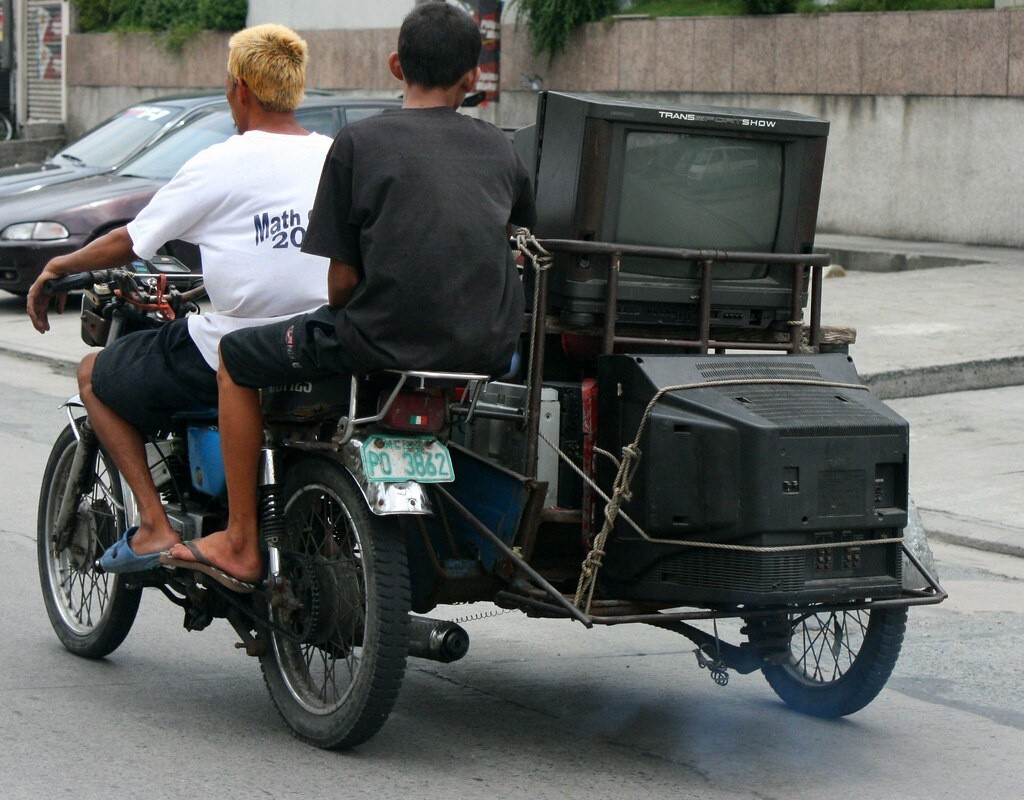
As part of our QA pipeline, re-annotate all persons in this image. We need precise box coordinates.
[157,2,539,594]
[26,23,336,576]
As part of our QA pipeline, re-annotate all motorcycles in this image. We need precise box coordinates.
[30,87,950,746]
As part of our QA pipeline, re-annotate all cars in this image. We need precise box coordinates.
[0,98,408,298]
[1,85,335,198]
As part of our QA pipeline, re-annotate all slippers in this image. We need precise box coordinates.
[159,538,256,594]
[100,525,159,573]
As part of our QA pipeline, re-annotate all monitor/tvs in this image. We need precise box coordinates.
[511,89,830,330]
[601,351,910,609]
